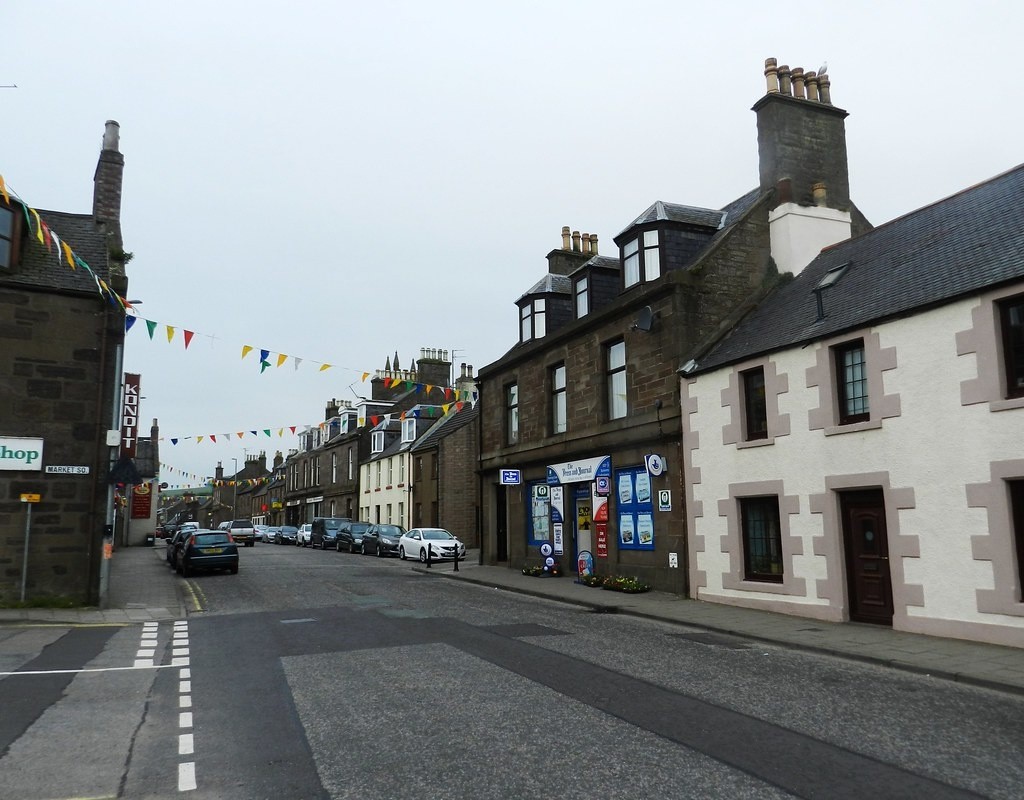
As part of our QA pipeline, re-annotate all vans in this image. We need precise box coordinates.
[312,517,352,549]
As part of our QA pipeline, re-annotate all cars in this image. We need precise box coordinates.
[218,521,229,529]
[276,526,299,544]
[263,527,279,543]
[335,520,374,553]
[253,525,269,542]
[156,527,164,537]
[164,525,239,576]
[296,523,313,547]
[361,524,408,557]
[399,528,466,563]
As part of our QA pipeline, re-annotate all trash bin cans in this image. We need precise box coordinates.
[146,533,155,547]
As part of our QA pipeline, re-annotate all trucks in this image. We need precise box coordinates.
[184,522,199,529]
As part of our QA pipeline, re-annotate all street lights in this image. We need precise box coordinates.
[231,458,237,519]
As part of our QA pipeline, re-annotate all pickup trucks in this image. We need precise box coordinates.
[226,519,255,547]
